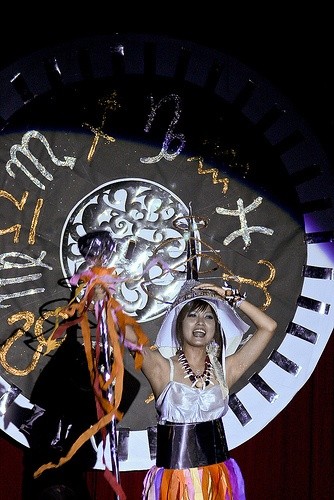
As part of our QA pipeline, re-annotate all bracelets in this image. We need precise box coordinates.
[224,289,248,309]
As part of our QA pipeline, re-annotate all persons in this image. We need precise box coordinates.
[94,281,277,500]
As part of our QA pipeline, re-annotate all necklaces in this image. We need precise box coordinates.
[175,349,215,390]
